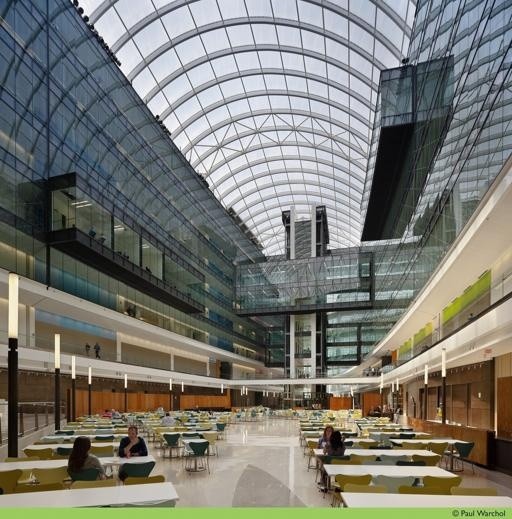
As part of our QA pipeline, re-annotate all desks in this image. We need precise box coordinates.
[0,407,512,508]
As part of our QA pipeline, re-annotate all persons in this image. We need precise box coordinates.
[118,425,148,482]
[85,342,91,358]
[127,303,133,316]
[94,342,101,359]
[111,408,120,417]
[319,430,346,494]
[146,266,152,273]
[66,435,107,481]
[103,409,112,416]
[317,425,346,449]
[160,412,174,426]
[366,365,381,377]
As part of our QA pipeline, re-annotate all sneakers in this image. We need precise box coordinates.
[321,486,328,493]
